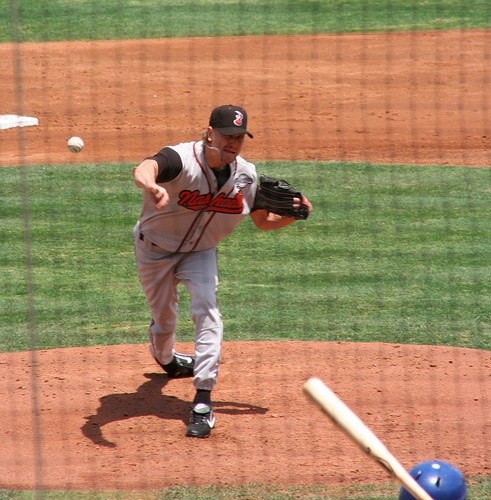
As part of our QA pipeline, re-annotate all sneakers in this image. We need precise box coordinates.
[185,402,216,439]
[156,350,197,378]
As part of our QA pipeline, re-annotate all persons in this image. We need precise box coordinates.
[134,104,312,438]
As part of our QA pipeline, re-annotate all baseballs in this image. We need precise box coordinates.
[68,137,84,154]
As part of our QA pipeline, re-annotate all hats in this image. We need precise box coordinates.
[209,104,254,140]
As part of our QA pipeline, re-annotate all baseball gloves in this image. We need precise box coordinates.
[251,173,310,223]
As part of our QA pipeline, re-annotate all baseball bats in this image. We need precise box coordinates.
[301,376,432,500]
